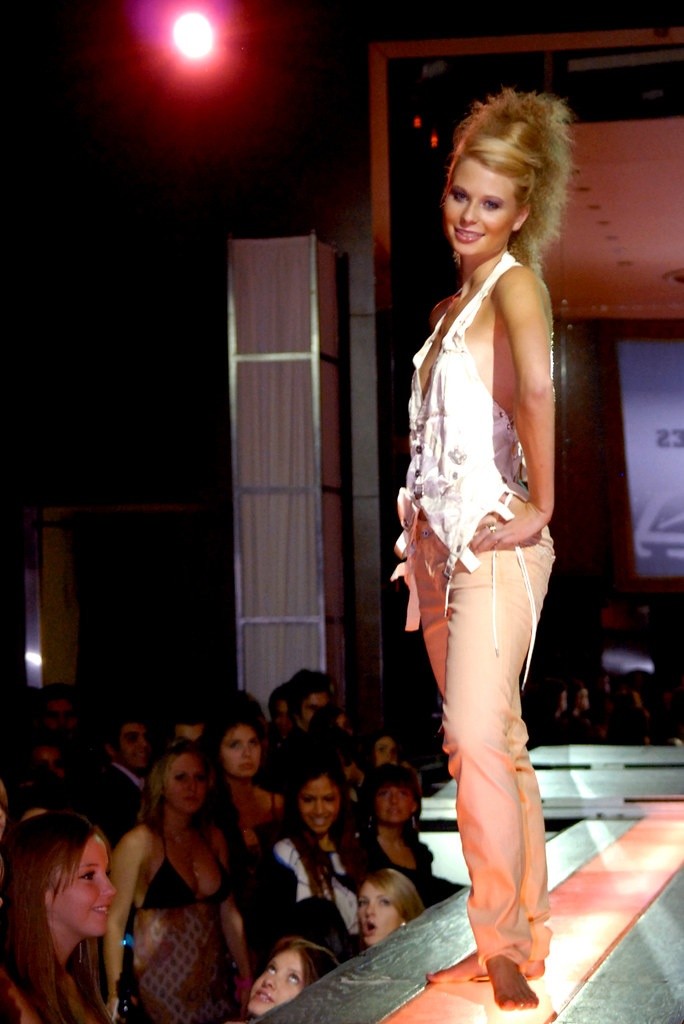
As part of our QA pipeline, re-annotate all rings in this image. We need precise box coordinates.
[489,524,496,533]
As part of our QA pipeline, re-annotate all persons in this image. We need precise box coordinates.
[0,665,436,1023]
[519,665,684,751]
[389,87,575,1011]
